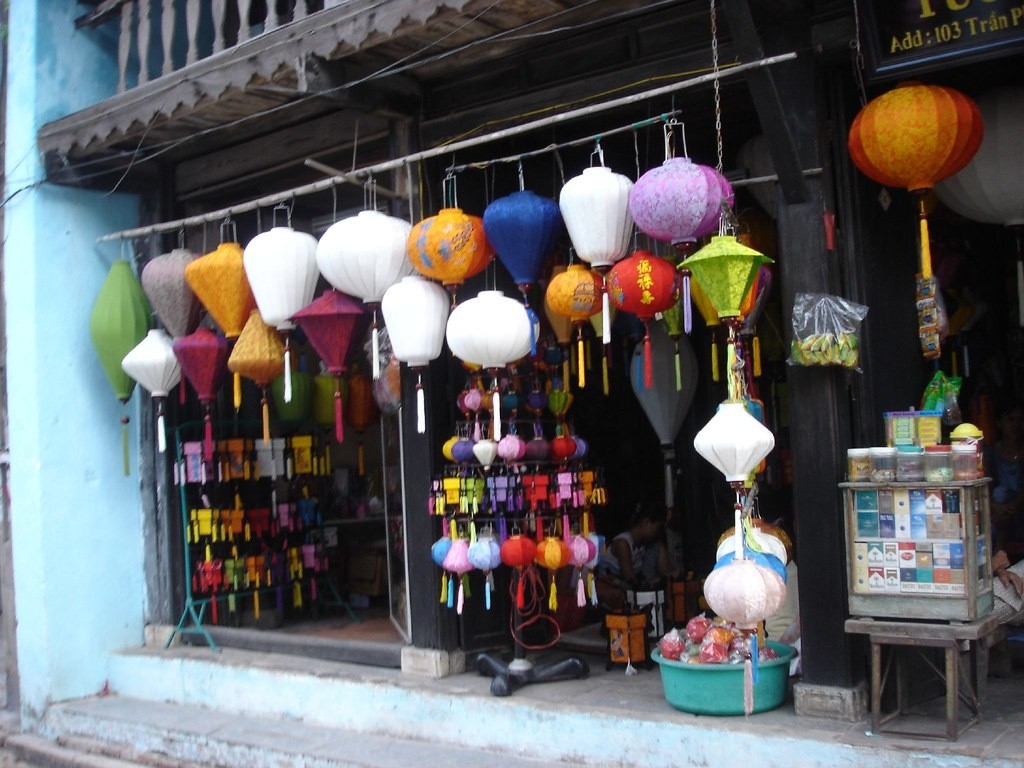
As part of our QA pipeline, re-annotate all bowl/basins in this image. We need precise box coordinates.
[651,640,798,715]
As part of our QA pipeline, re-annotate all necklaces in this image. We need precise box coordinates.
[1003,442,1020,462]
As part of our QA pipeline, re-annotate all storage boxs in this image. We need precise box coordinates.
[852,490,987,584]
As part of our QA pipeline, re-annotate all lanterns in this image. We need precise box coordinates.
[931,75,1024,327]
[88,156,793,715]
[924,241,992,381]
[847,80,982,279]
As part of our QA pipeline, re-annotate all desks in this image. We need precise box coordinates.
[322,512,369,525]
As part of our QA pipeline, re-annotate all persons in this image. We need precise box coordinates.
[596,508,673,640]
[983,401,1024,598]
